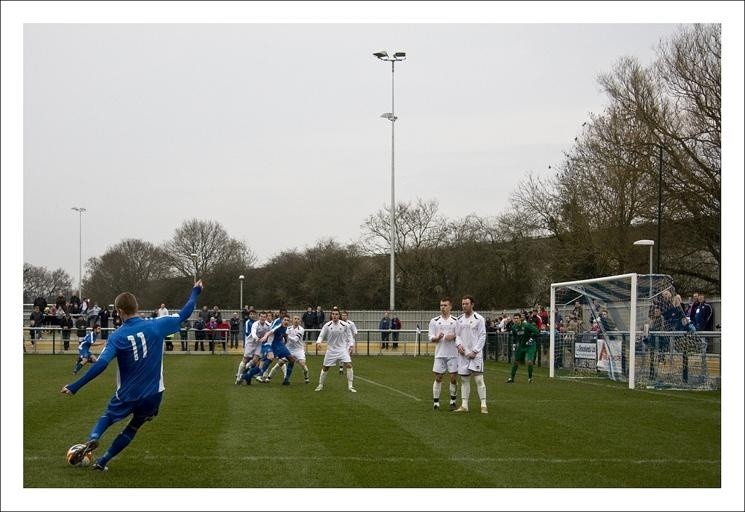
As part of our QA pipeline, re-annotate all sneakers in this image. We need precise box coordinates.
[235,370,358,393]
[504,377,514,383]
[453,405,469,413]
[69,438,100,466]
[527,378,535,384]
[449,402,456,411]
[480,406,488,414]
[91,464,109,472]
[433,404,439,411]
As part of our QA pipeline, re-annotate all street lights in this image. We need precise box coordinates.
[633,238,656,301]
[69,203,89,307]
[189,250,200,310]
[238,273,245,310]
[372,47,409,311]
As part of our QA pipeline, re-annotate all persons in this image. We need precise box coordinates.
[484,285,714,383]
[454,295,489,415]
[427,295,459,410]
[61,279,204,471]
[27,288,324,388]
[377,312,391,349]
[339,312,358,375]
[329,305,342,320]
[392,313,402,348]
[315,309,357,393]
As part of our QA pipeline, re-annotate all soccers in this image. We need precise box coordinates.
[68,444,92,467]
